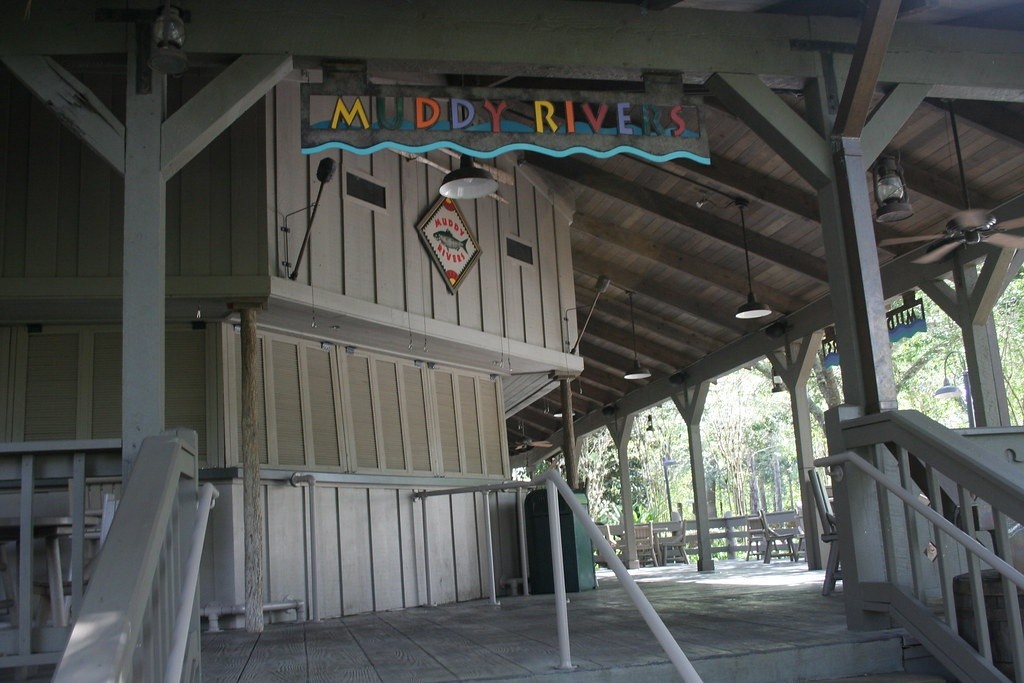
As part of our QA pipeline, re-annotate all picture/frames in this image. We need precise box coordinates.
[416,196,483,296]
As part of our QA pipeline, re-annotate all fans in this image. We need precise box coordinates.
[878,101,1024,265]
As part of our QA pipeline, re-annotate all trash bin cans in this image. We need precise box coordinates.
[524,490,598,596]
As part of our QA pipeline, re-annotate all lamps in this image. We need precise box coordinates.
[771,364,789,393]
[515,415,532,444]
[439,154,499,199]
[734,198,772,319]
[870,150,914,222]
[554,407,575,418]
[623,290,651,380]
[935,352,968,399]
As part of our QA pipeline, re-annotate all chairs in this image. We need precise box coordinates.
[745,510,799,564]
[595,520,691,567]
[807,469,845,596]
